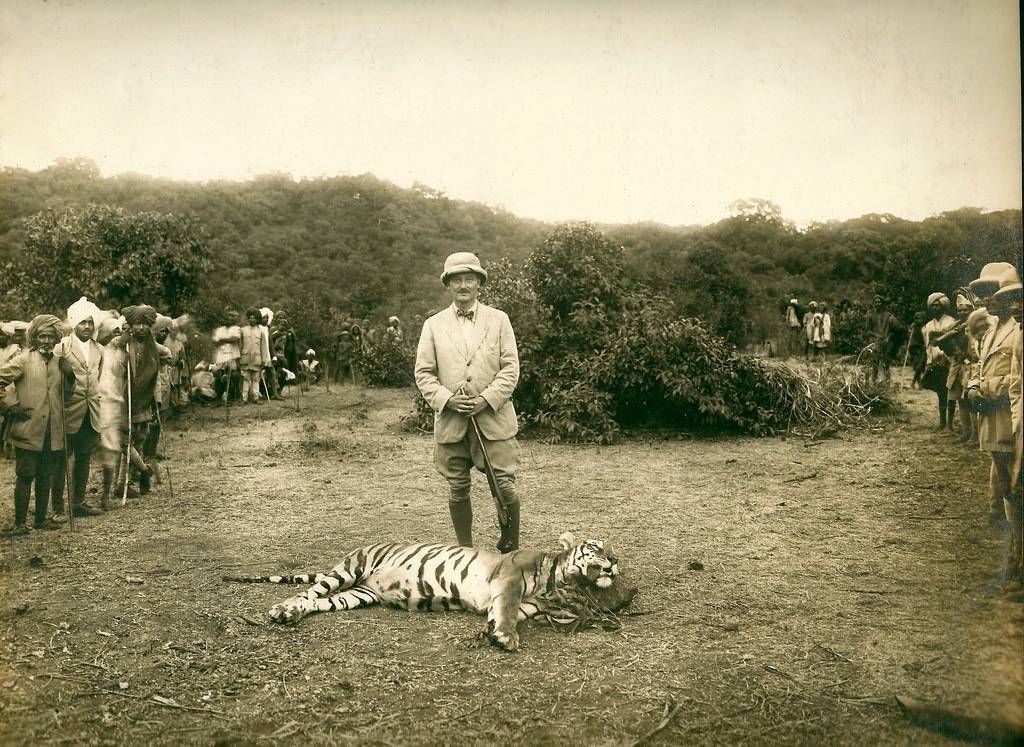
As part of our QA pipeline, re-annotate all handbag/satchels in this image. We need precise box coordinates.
[921,362,948,390]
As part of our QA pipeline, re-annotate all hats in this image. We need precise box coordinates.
[994,266,1022,296]
[441,252,487,288]
[969,262,1016,295]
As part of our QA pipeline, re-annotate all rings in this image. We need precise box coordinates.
[457,408,461,413]
[979,403,982,407]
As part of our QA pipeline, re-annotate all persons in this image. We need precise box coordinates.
[332,319,378,386]
[97,322,112,346]
[382,316,403,346]
[211,310,241,408]
[854,295,927,390]
[414,252,520,555]
[269,311,297,400]
[189,362,217,403]
[0,314,76,535]
[237,307,268,406]
[163,314,191,413]
[786,299,852,361]
[300,349,322,385]
[118,315,128,330]
[107,319,122,337]
[921,261,1024,593]
[257,307,285,401]
[52,295,104,522]
[413,315,422,326]
[0,320,28,453]
[99,302,173,510]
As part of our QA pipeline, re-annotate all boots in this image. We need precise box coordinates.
[499,497,519,552]
[447,498,472,546]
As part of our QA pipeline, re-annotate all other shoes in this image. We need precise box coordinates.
[271,394,284,400]
[956,432,969,441]
[918,384,923,390]
[985,513,1002,523]
[139,465,154,495]
[33,519,61,531]
[1005,556,1024,621]
[932,424,945,433]
[910,382,915,389]
[115,485,136,498]
[131,470,142,480]
[51,510,68,524]
[72,502,102,517]
[143,452,166,462]
[13,524,29,536]
[101,495,117,511]
[239,400,247,406]
[968,435,977,443]
[254,398,263,405]
[947,425,953,432]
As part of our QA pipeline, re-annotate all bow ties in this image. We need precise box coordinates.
[457,309,474,321]
